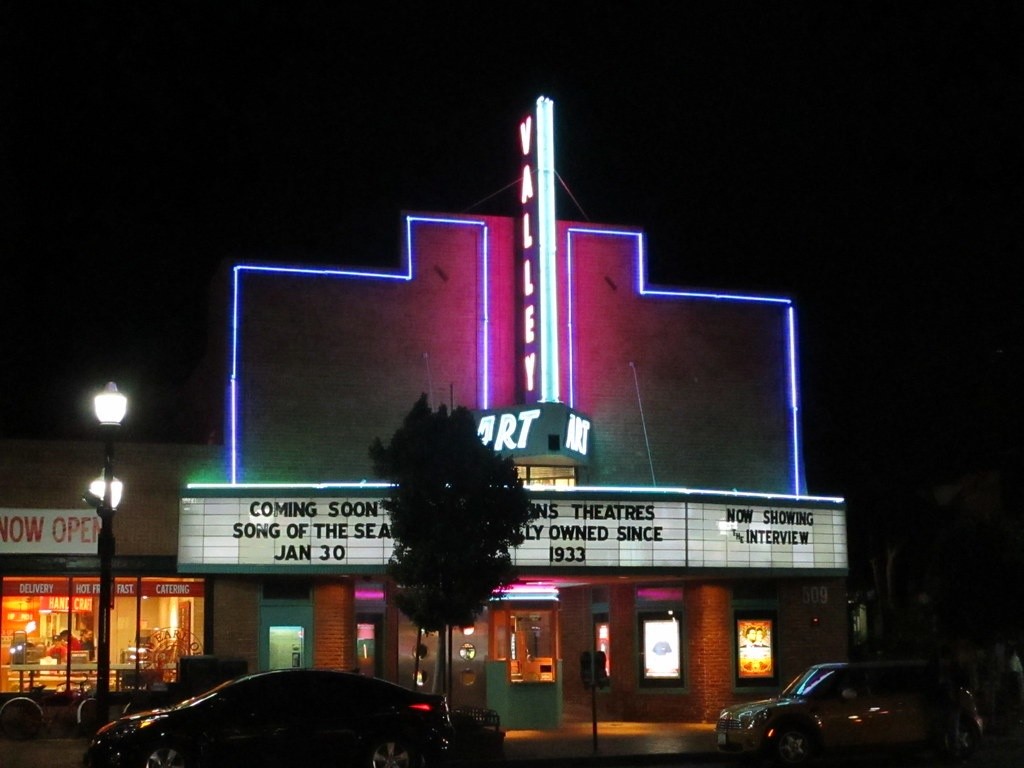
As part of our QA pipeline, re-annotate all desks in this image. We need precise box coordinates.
[10,663,176,693]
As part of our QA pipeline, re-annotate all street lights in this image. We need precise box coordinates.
[89,379,133,722]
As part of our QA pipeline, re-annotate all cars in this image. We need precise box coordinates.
[81,666,459,768]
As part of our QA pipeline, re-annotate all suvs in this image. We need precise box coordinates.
[713,662,987,768]
